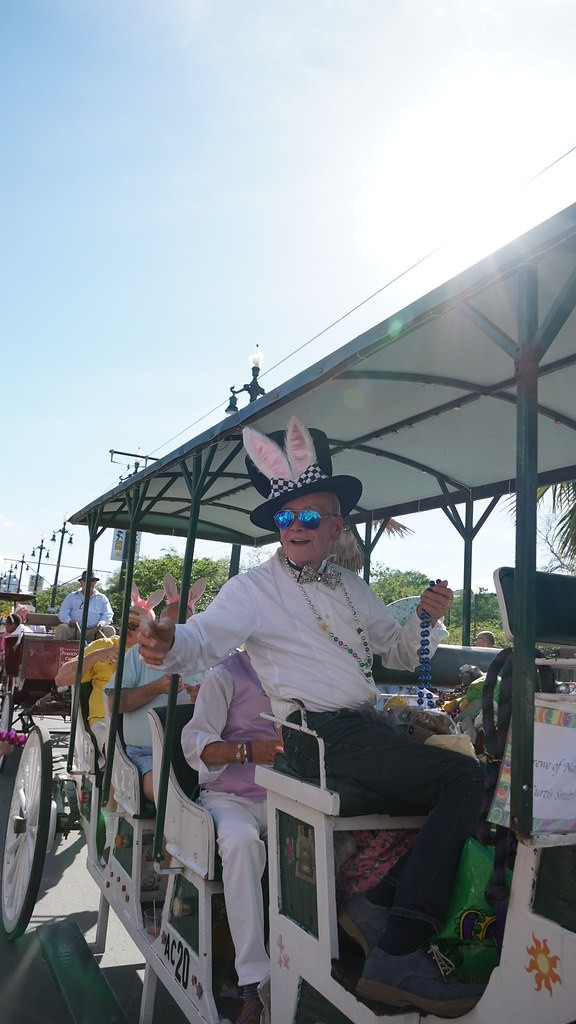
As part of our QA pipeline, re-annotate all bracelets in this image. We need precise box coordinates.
[236,740,253,765]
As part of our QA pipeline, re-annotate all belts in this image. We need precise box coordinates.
[283,712,329,741]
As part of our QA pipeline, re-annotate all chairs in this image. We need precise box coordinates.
[0,567,576,1022]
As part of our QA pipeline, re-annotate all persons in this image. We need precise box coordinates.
[104,602,207,803]
[55,571,116,639]
[137,491,500,1018]
[444,664,502,758]
[0,605,46,659]
[55,606,155,812]
[475,631,494,648]
[179,651,285,1024]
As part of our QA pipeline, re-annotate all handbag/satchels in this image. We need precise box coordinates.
[434,837,516,983]
[387,706,451,742]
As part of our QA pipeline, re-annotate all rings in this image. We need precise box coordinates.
[138,645,144,652]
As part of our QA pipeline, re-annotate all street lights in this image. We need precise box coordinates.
[119,446,144,594]
[14,555,29,594]
[31,536,49,595]
[0,564,16,593]
[48,516,73,614]
[225,344,267,581]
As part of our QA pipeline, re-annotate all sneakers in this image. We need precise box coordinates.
[338,892,390,958]
[357,943,487,1018]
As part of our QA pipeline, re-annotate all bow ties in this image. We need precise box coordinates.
[287,556,342,590]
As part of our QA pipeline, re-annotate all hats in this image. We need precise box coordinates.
[129,606,155,622]
[242,415,363,532]
[386,597,448,642]
[78,571,100,581]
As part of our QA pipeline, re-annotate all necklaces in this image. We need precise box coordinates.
[285,556,373,680]
[417,579,443,709]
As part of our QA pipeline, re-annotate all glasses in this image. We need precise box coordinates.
[473,638,487,642]
[273,508,339,530]
[118,621,139,631]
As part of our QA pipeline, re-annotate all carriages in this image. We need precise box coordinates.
[0,195,576,1024]
[0,589,119,772]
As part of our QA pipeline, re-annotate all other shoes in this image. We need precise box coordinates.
[173,899,192,919]
[235,997,263,1024]
